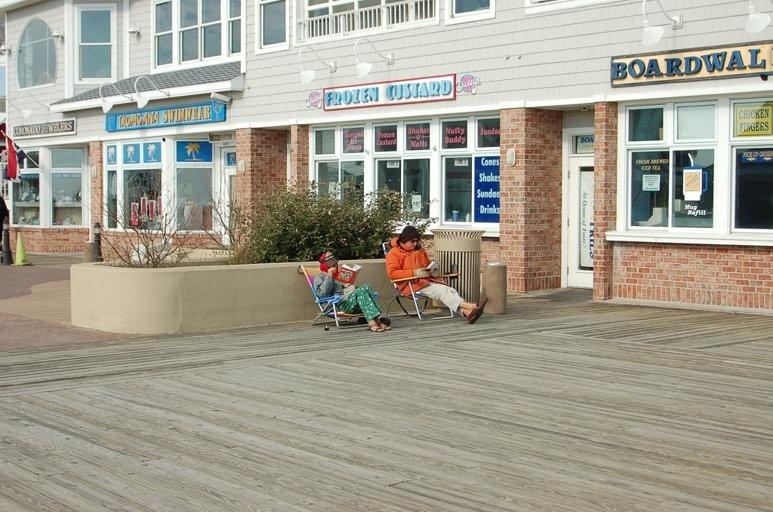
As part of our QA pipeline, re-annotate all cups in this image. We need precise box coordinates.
[451,210,461,222]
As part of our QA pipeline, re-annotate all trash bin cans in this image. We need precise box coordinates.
[83,241,95,262]
[482,262,507,315]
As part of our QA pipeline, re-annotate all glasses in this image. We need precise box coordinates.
[410,239,419,243]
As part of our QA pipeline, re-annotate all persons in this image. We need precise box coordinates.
[385,226,487,324]
[311,250,393,334]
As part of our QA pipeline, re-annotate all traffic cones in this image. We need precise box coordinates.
[11,232,33,266]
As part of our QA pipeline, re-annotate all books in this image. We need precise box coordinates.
[425,260,440,274]
[333,263,363,288]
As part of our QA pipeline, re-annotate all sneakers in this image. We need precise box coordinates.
[471,296,488,316]
[467,306,484,324]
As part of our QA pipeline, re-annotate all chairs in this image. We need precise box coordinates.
[296,258,387,331]
[379,240,464,326]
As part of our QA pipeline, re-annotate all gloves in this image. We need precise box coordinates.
[431,268,439,277]
[414,268,431,278]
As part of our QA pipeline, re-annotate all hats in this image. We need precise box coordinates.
[318,250,338,268]
[399,225,421,244]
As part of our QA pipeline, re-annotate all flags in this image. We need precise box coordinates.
[0,131,29,185]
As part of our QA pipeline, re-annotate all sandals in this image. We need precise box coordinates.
[378,322,392,330]
[368,324,385,332]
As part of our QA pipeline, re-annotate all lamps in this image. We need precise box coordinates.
[127,22,141,37]
[294,42,337,86]
[132,74,173,110]
[744,0,772,35]
[97,78,130,117]
[637,0,682,47]
[349,35,395,80]
[48,28,65,42]
[5,85,51,120]
[0,40,10,57]
